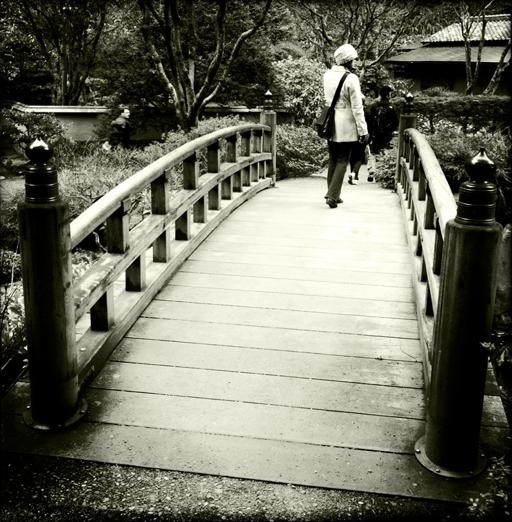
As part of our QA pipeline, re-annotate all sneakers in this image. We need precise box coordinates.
[324,168,374,208]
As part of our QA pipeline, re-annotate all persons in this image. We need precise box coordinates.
[102,101,133,150]
[364,84,400,182]
[347,96,376,186]
[321,41,371,211]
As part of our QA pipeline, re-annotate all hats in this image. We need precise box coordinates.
[334,44,358,66]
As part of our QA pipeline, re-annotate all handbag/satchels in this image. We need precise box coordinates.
[315,106,335,138]
